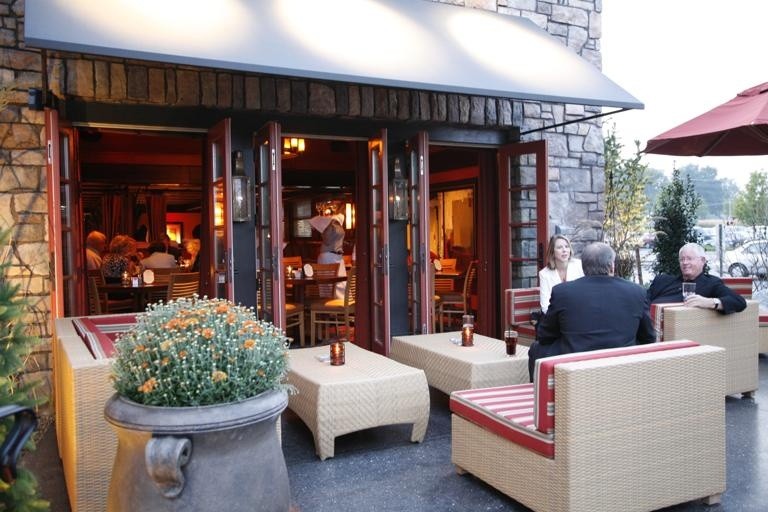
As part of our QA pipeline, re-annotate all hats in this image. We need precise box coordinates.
[715,240,768,279]
[644,233,658,250]
[690,226,768,249]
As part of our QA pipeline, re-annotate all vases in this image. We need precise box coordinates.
[103,291,301,409]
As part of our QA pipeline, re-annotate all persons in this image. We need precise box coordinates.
[646,242,747,315]
[537,234,585,316]
[84,211,354,303]
[527,241,657,383]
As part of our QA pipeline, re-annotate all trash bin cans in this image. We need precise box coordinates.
[710,297,720,310]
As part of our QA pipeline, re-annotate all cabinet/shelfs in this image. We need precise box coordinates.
[121,272,141,286]
[284,264,314,279]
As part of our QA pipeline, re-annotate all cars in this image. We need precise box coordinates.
[682,282,695,303]
[330,341,345,365]
[504,330,517,358]
[462,314,474,345]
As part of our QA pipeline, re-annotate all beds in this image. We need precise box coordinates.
[643,80,767,155]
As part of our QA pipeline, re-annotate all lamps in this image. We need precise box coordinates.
[447,338,730,512]
[55,330,149,512]
[644,294,761,400]
[716,274,768,358]
[166,270,201,302]
[145,266,185,283]
[63,272,103,318]
[503,285,547,347]
[247,246,479,349]
[51,309,147,459]
[84,267,113,314]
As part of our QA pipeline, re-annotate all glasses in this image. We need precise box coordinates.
[389,157,411,223]
[229,149,252,224]
[279,137,308,158]
